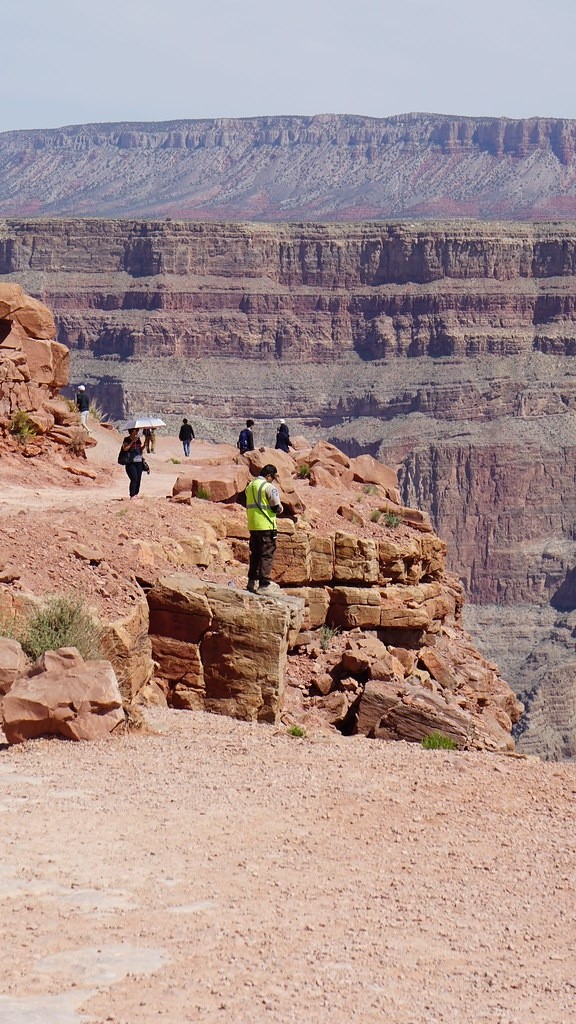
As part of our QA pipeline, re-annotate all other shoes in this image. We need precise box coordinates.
[246,580,260,591]
[256,585,282,597]
[147,450,150,453]
[151,449,154,451]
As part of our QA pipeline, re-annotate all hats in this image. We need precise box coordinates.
[280,419,285,423]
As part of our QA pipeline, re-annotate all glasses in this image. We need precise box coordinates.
[270,474,275,480]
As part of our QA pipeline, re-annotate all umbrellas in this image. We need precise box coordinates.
[124,417,166,431]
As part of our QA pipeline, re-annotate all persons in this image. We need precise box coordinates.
[237,420,254,454]
[179,419,195,457]
[143,429,157,454]
[245,464,284,589]
[275,419,293,452]
[118,428,150,499]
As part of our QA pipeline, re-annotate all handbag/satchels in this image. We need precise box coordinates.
[118,447,128,465]
